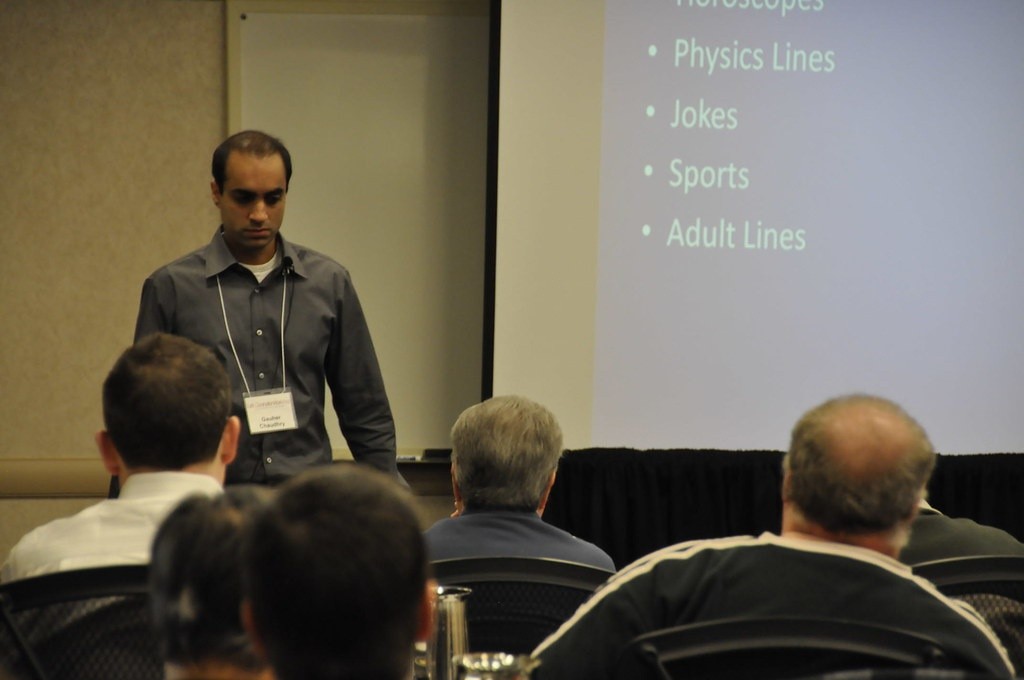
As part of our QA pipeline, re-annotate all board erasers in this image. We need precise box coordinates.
[421,447,453,461]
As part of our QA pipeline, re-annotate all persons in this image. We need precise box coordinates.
[0,332,241,584]
[132,130,399,486]
[148,465,432,680]
[528,395,1024,680]
[421,395,616,573]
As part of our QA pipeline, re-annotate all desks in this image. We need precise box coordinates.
[542,448,1024,572]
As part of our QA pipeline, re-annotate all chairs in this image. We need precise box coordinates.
[618,614,992,680]
[428,556,615,656]
[0,564,164,680]
[911,553,1024,680]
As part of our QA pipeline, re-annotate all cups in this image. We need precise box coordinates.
[426,585,474,680]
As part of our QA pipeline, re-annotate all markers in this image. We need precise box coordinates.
[396,457,416,460]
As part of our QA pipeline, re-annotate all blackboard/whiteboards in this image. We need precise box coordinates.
[225,2,490,466]
